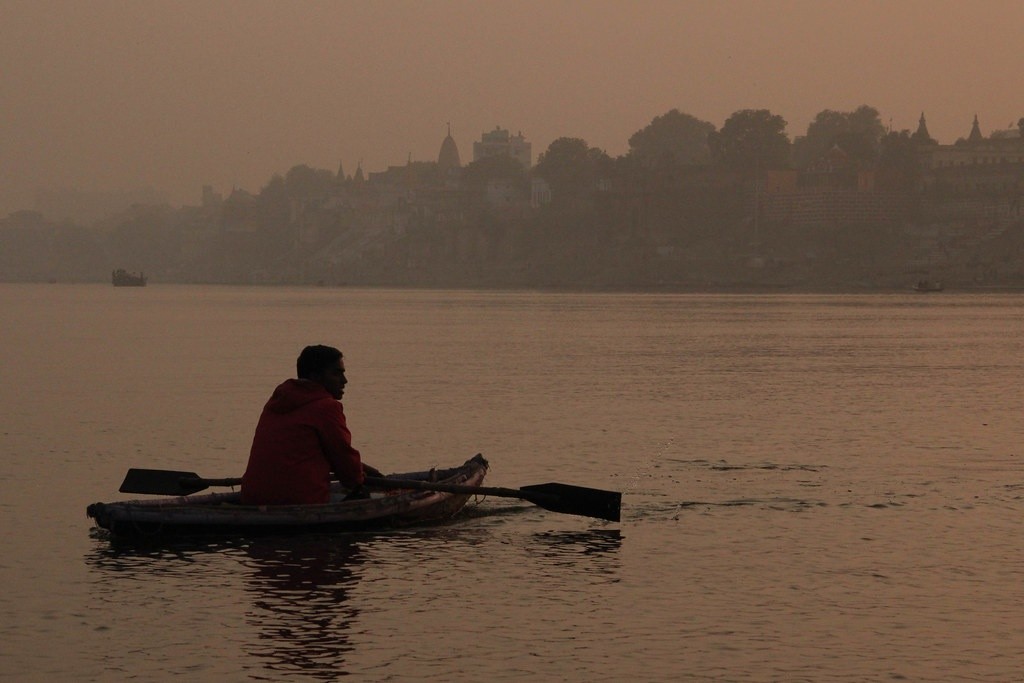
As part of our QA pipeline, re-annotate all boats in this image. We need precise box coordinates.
[112,268,148,286]
[911,285,940,291]
[86,453,492,536]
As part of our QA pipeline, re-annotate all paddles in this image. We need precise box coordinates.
[120,467,623,522]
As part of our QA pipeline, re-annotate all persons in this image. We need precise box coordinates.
[242,345,386,505]
